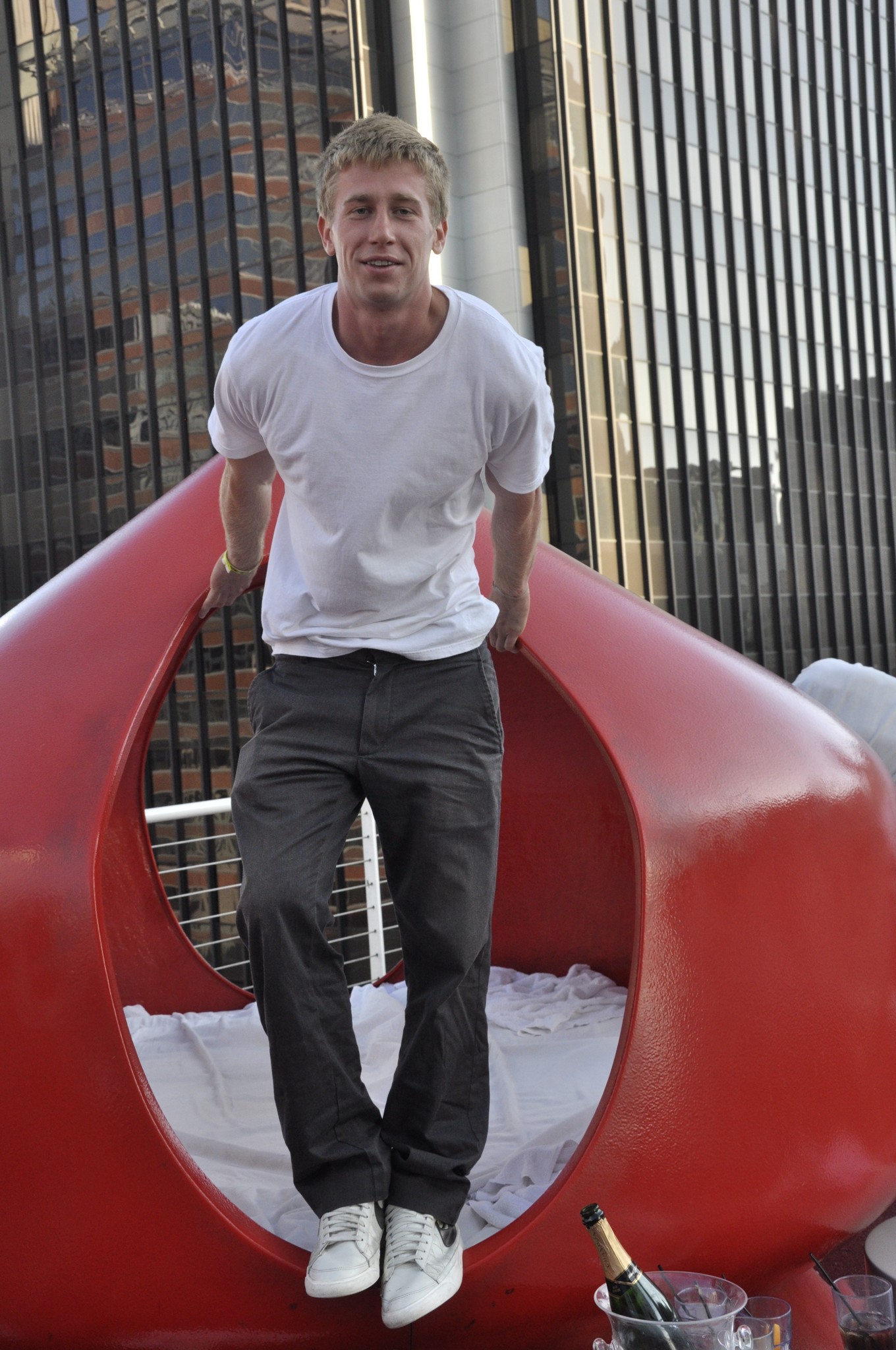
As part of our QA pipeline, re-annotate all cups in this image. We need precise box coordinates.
[733,1316,775,1349]
[738,1297,793,1350]
[831,1275,895,1349]
[675,1287,728,1328]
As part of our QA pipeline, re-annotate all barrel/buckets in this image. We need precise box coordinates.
[593,1272,747,1350]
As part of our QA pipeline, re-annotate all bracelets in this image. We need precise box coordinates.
[492,581,528,601]
[222,551,264,577]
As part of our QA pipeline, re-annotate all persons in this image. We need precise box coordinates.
[200,112,555,1330]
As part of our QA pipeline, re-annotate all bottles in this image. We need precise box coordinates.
[578,1202,697,1349]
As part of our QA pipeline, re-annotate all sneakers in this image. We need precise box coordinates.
[380,1204,464,1329]
[304,1200,384,1298]
